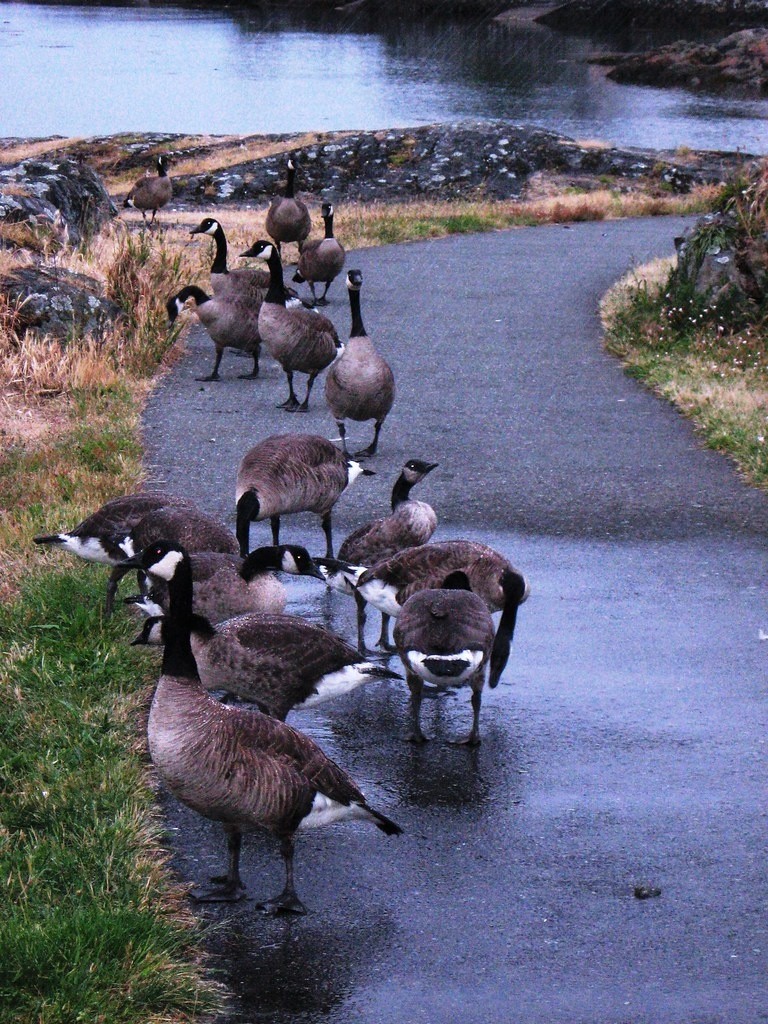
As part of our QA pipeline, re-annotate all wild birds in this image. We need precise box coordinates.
[30,431,532,918]
[120,154,173,227]
[163,152,397,461]
